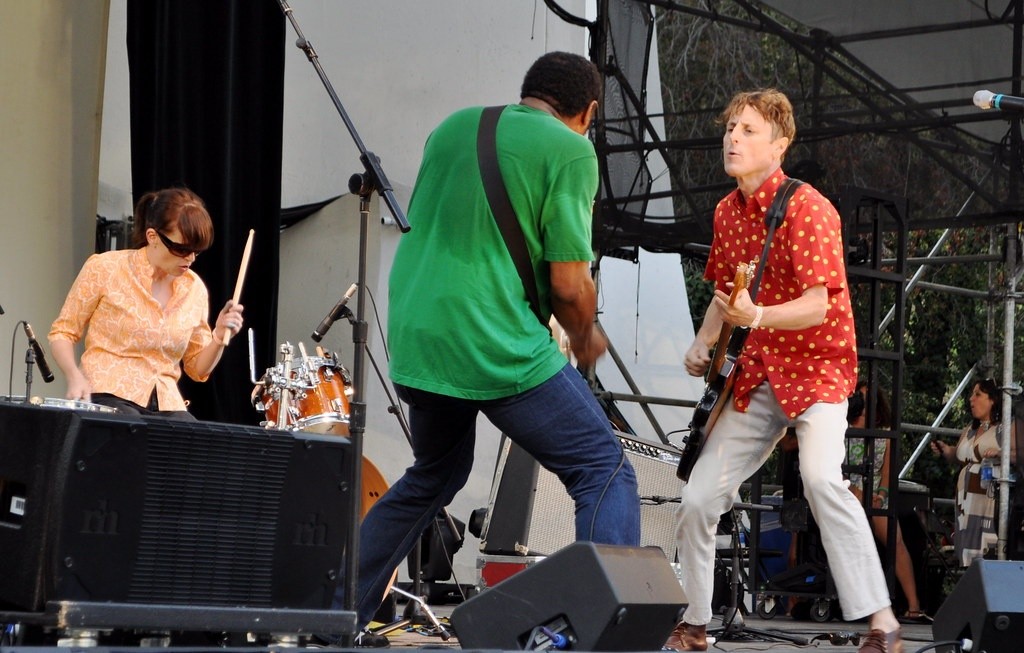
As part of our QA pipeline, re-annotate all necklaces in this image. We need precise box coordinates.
[979,421,991,431]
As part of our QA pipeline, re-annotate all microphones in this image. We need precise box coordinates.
[311,283,359,342]
[973,90,1024,111]
[24,321,55,383]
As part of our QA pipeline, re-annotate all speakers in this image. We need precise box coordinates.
[0,402,356,619]
[481,422,688,564]
[932,558,1024,653]
[450,540,690,652]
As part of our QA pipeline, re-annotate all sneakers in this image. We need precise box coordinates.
[310,626,391,648]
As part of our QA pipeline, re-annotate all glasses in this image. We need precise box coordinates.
[985,376,996,386]
[155,228,201,257]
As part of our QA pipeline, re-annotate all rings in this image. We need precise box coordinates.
[228,322,236,328]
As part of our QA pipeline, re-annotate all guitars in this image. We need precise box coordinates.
[676,255,759,483]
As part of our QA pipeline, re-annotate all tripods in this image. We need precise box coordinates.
[636,493,809,648]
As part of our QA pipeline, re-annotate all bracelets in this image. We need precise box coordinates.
[749,306,763,329]
[877,487,889,497]
[877,494,885,503]
[212,328,225,346]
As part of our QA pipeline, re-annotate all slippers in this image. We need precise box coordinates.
[904,610,920,618]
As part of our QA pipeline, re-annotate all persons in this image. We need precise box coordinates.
[778,380,920,618]
[930,380,1016,568]
[332,52,641,653]
[46,188,243,421]
[662,88,904,653]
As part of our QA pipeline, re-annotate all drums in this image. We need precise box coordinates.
[250,355,353,437]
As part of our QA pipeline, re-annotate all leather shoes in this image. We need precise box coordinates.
[664,622,708,651]
[856,626,904,653]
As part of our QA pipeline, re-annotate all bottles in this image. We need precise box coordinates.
[731,520,745,549]
[980,457,993,488]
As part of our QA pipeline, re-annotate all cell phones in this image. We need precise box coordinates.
[931,438,944,455]
